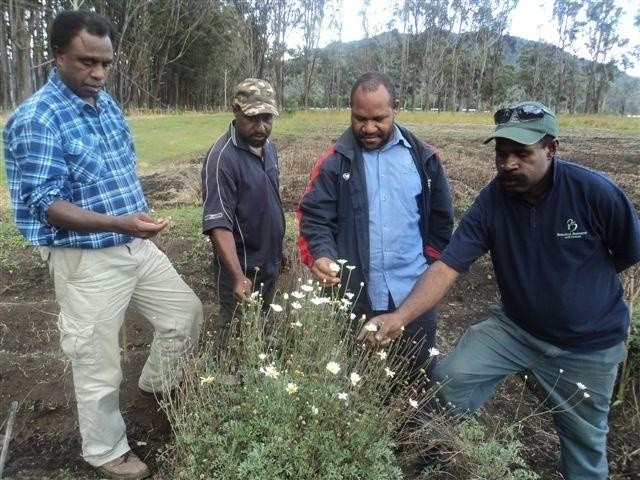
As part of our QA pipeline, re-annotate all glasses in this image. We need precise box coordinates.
[493,104,557,126]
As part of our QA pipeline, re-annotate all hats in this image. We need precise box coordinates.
[233,78,279,117]
[482,100,558,145]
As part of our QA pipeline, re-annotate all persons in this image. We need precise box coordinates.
[357,101,640,480]
[201,77,285,361]
[298,71,454,432]
[3,8,204,479]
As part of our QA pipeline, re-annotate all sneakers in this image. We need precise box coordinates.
[96,449,151,480]
